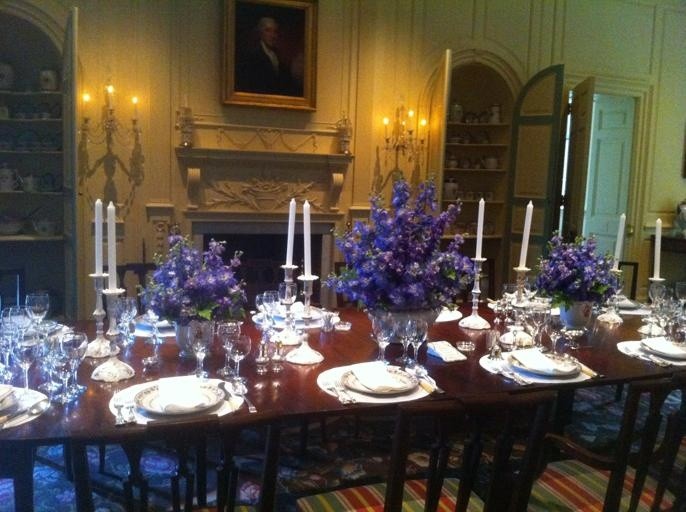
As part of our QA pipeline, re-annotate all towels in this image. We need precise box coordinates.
[511,345,579,375]
[158,372,205,411]
[280,298,309,319]
[644,335,685,358]
[351,359,410,393]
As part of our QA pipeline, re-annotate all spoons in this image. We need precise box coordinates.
[1,399,51,430]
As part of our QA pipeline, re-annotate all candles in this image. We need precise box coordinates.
[639,217,666,335]
[458,198,490,329]
[270,198,304,345]
[285,200,324,363]
[91,200,136,382]
[84,199,121,358]
[513,200,534,304]
[597,212,626,323]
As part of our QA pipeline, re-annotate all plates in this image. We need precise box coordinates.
[340,366,421,397]
[508,351,582,377]
[640,337,685,361]
[134,381,226,414]
[0,383,53,431]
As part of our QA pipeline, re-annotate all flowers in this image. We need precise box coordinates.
[527,230,624,312]
[136,234,247,324]
[320,172,487,312]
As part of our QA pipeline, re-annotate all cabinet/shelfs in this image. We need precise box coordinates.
[441,122,511,299]
[0,91,65,318]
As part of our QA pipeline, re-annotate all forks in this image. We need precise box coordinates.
[230,380,257,413]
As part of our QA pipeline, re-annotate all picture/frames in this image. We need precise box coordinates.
[222,0,318,111]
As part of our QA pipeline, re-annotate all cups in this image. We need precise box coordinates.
[675,280,685,310]
[543,317,568,353]
[255,293,274,332]
[9,332,40,396]
[60,332,88,396]
[112,297,138,347]
[457,325,479,352]
[531,303,547,338]
[263,290,280,313]
[186,319,215,378]
[227,333,252,385]
[372,316,396,367]
[278,282,298,312]
[392,315,417,366]
[35,336,66,394]
[405,319,428,368]
[8,305,33,339]
[24,292,50,332]
[648,283,667,316]
[217,319,241,378]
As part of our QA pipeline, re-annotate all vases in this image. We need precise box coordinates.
[176,323,213,358]
[561,301,592,330]
[380,311,435,344]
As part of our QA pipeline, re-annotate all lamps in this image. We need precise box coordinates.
[378,106,422,171]
[78,84,146,187]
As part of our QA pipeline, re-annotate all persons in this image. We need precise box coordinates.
[248,17,300,97]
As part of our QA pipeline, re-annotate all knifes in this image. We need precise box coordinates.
[215,381,237,412]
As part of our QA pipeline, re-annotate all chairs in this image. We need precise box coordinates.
[509,372,686,512]
[450,259,494,302]
[569,261,638,412]
[71,415,281,512]
[320,260,392,444]
[277,391,557,512]
[99,263,157,471]
[0,267,76,482]
[0,270,106,482]
